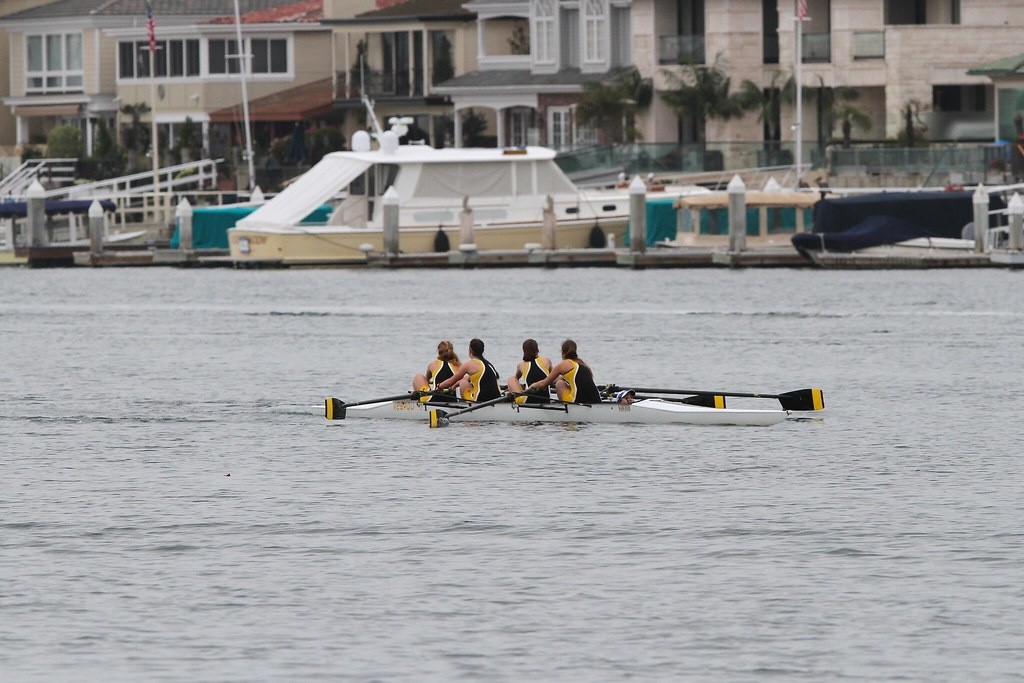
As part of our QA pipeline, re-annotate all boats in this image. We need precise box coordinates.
[308,394,794,426]
[0,155,120,215]
[791,183,1001,267]
[225,54,713,271]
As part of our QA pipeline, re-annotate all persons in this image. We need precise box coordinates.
[436,339,503,407]
[526,340,602,403]
[617,387,635,407]
[412,341,467,404]
[507,340,554,409]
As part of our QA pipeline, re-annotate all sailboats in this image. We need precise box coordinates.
[623,1,833,249]
[170,0,337,250]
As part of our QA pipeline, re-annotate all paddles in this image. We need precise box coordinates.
[546,386,727,410]
[324,387,450,421]
[428,388,534,429]
[493,383,827,411]
